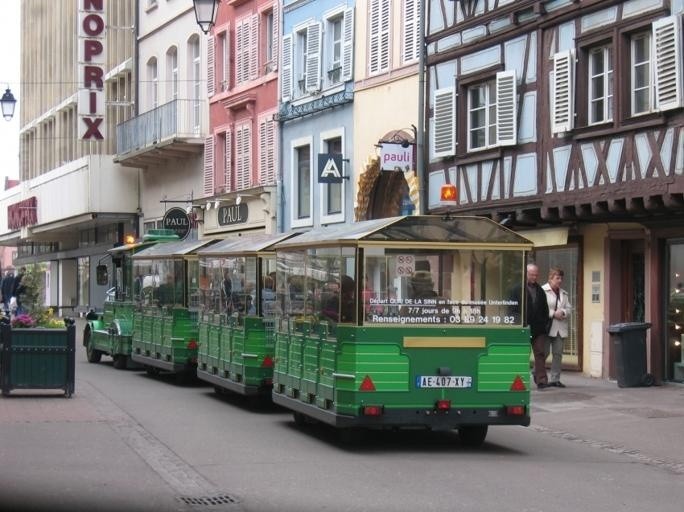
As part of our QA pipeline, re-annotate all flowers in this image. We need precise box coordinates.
[0,258,66,329]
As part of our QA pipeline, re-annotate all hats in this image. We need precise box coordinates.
[407,271,434,286]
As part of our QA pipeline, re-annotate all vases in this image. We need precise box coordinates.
[0,316,76,399]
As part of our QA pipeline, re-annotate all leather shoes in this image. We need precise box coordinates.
[550,381,565,387]
[537,383,549,387]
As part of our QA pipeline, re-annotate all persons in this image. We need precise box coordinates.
[541,268,573,388]
[223,271,276,315]
[321,274,385,324]
[401,270,443,321]
[159,272,174,303]
[0,271,15,311]
[10,272,18,310]
[11,267,30,315]
[508,263,549,389]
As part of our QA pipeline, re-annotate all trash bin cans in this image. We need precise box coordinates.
[606,321,655,389]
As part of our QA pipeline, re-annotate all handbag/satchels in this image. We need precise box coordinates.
[545,318,553,333]
